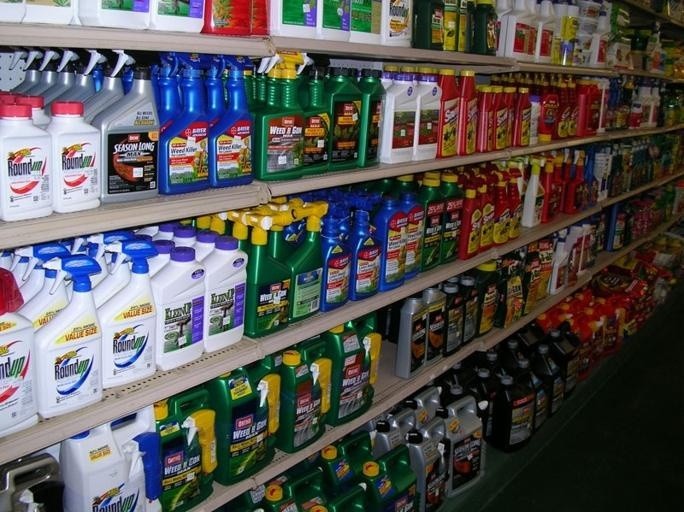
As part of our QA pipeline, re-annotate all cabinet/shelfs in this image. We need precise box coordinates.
[0,20,683,511]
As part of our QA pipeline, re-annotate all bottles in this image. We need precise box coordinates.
[0,0,613,66]
[553,203,628,294]
[369,167,553,381]
[302,66,684,176]
[150,221,248,373]
[0,90,103,223]
[535,289,625,384]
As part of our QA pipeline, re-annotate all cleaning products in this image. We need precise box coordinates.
[1,0,684,512]
